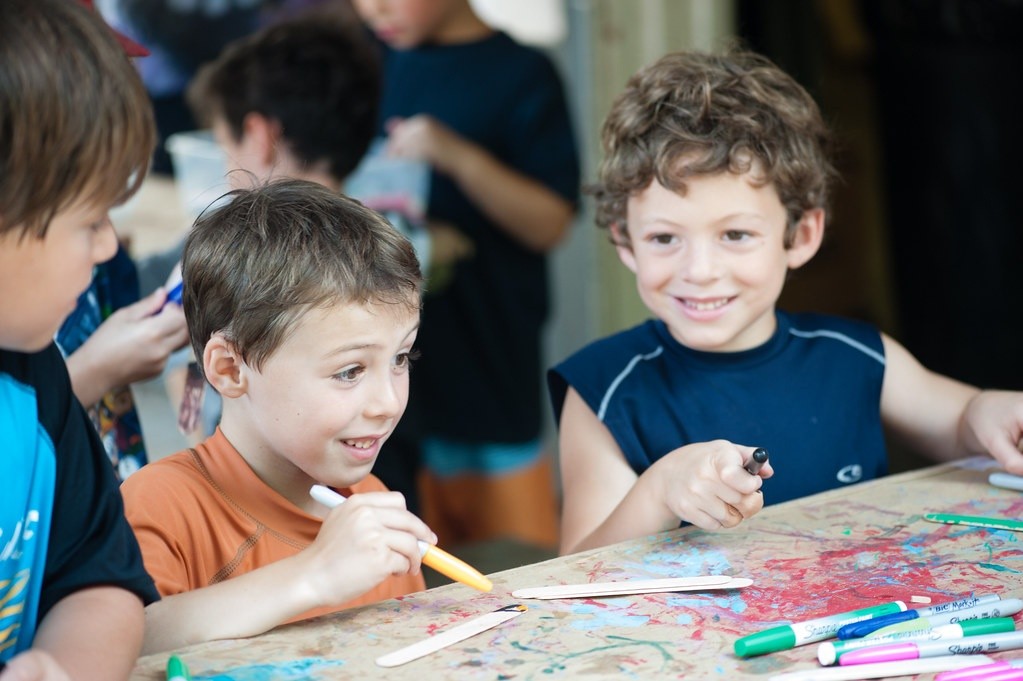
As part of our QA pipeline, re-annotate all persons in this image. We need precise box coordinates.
[1,0,159,681]
[97,0,583,556]
[118,180,438,659]
[556,50,1021,555]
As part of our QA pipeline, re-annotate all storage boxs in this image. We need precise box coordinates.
[167,127,428,234]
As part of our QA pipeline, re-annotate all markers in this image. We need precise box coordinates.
[309,481,494,594]
[745,447,768,476]
[165,654,187,681]
[731,592,1023,681]
[988,472,1023,492]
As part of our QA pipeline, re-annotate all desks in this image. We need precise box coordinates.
[136,452,1023,681]
